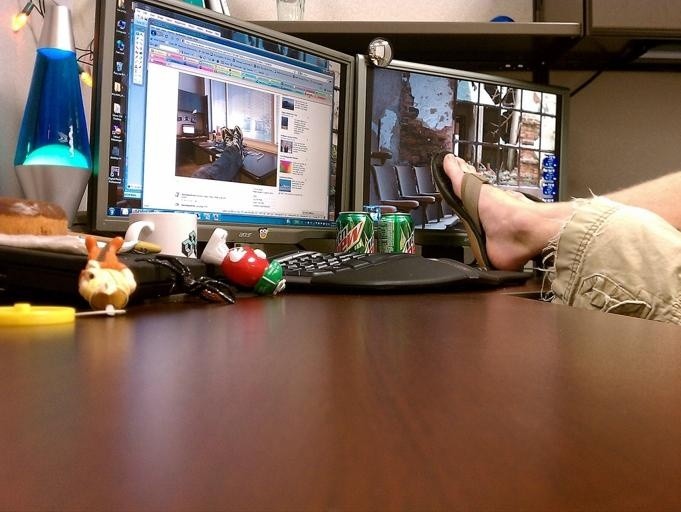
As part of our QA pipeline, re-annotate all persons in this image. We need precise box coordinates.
[191,126,242,181]
[434,151,681,332]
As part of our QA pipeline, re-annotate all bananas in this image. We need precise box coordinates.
[267,250,534,296]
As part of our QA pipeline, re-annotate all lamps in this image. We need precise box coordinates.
[412,166,454,218]
[372,165,435,230]
[396,165,442,224]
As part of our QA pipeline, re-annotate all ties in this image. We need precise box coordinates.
[85,4,355,242]
[356,52,571,262]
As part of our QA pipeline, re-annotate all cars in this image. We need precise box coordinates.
[123,211,197,259]
[276,0,305,21]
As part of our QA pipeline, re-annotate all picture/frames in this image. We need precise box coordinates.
[191,141,277,185]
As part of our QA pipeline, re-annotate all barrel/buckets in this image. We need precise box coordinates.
[432,151,494,273]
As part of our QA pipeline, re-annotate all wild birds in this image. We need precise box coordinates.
[181,123,197,139]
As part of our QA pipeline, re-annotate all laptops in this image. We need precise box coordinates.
[1,199,67,236]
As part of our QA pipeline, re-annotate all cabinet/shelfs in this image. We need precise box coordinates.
[336,210,375,255]
[377,212,416,256]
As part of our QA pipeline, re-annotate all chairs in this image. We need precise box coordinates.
[221,125,232,147]
[233,126,243,159]
